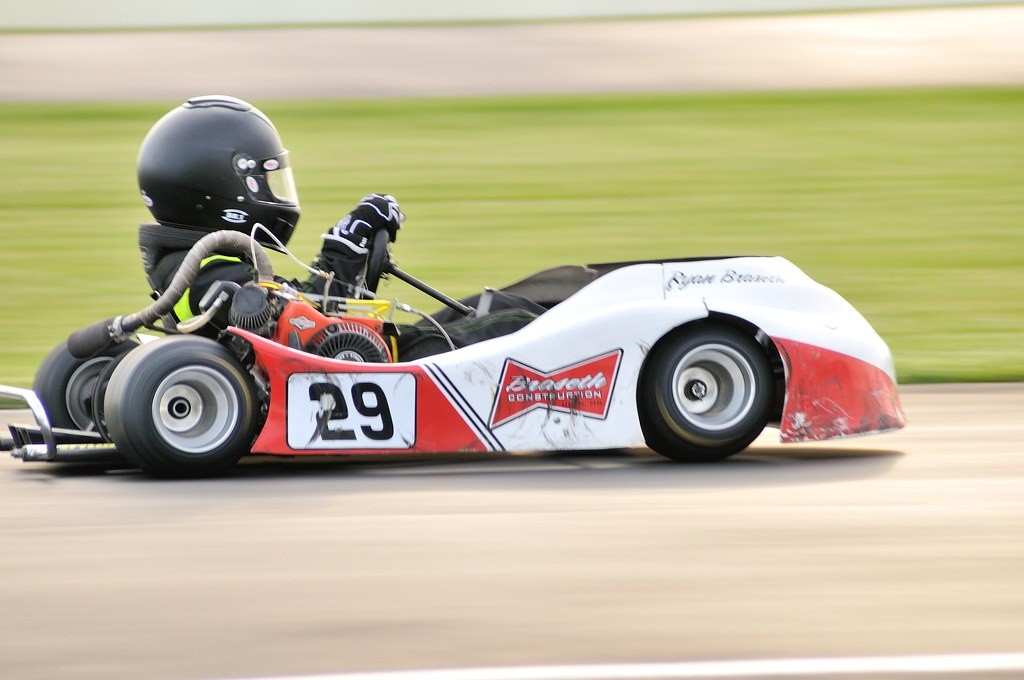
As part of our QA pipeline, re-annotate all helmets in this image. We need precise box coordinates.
[137,96,300,248]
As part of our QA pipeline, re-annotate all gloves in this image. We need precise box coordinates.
[322,194,403,259]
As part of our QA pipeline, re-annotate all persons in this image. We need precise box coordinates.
[137,95,540,364]
